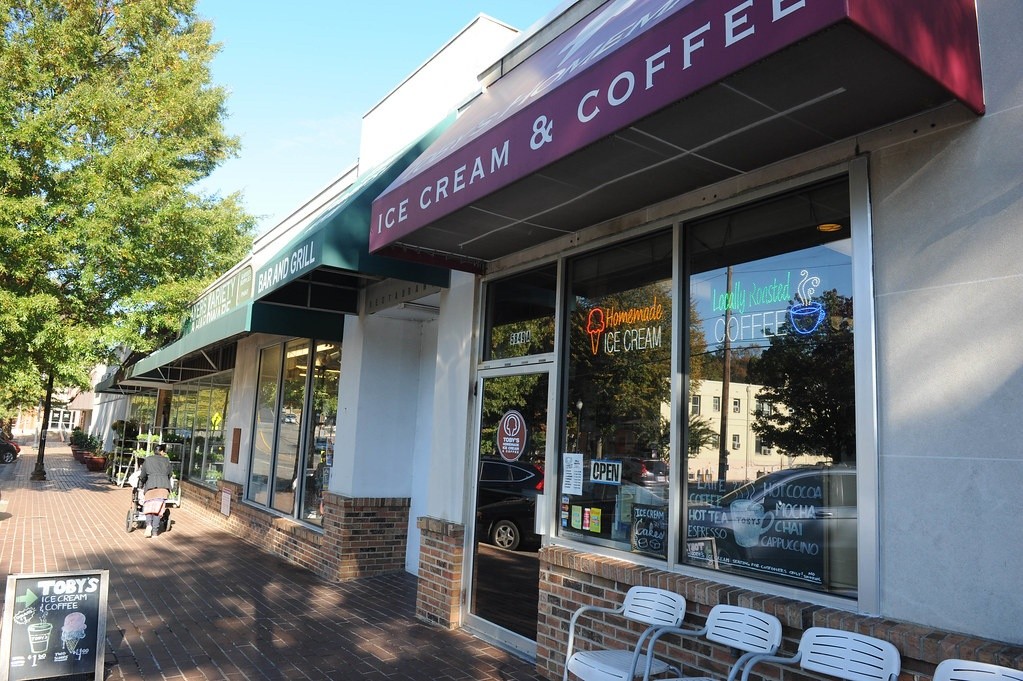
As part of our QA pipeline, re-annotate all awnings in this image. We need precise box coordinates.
[67,389,92,411]
[254,0,987,316]
[95,373,157,397]
[121,302,345,385]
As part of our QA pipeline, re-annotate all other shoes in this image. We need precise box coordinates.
[152,532,158,538]
[144,525,152,538]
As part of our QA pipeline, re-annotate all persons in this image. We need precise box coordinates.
[139,445,173,537]
[307,452,333,519]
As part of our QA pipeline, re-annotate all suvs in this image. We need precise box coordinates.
[652,462,857,589]
[478,458,543,511]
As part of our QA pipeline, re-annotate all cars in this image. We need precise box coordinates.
[477,479,670,551]
[284,414,297,423]
[315,437,335,453]
[0,436,16,463]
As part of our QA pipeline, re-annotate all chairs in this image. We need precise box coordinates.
[563,586,1022,681]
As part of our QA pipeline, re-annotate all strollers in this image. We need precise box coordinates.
[125,475,171,532]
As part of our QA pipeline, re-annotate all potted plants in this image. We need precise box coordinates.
[69,426,106,472]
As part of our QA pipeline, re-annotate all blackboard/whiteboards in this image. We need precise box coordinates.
[0,569,109,681]
[629,501,668,561]
[680,464,831,593]
